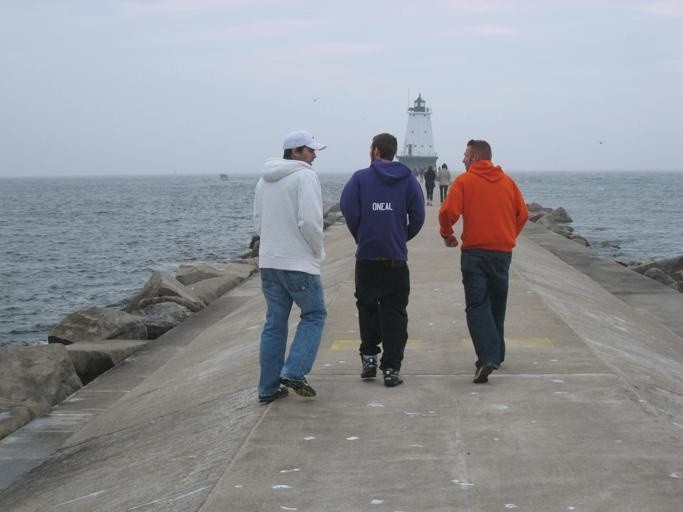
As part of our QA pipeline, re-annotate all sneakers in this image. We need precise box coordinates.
[472,361,495,385]
[359,354,378,379]
[279,376,318,399]
[383,368,403,387]
[258,386,289,404]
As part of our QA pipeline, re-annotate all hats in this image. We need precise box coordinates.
[282,130,327,155]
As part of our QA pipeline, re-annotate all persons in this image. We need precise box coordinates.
[339,133,425,386]
[253,132,327,405]
[413,163,451,206]
[438,139,528,383]
